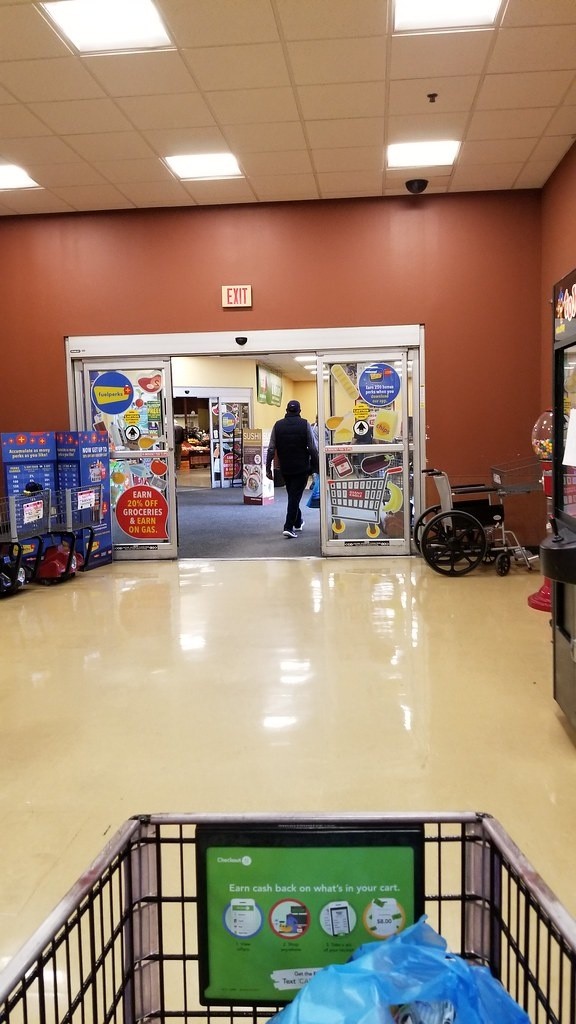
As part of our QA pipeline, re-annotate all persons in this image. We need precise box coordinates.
[265,400,319,538]
[309,413,330,490]
[173,419,183,471]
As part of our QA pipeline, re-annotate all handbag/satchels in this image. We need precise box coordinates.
[265,915,532,1024]
[307,473,320,508]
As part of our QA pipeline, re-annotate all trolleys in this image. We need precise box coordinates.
[328,467,402,539]
[353,453,413,501]
[0,813,576,1024]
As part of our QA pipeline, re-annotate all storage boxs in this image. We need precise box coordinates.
[180,460,189,470]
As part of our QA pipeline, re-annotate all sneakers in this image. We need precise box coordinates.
[283,529,297,537]
[296,519,304,530]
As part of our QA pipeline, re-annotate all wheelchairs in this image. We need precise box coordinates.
[414,456,542,577]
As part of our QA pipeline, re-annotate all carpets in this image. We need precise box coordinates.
[112,488,415,558]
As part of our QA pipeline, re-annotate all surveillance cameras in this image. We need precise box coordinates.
[406,178,429,193]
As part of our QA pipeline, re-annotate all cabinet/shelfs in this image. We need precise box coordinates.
[180,428,210,468]
[540,269,576,726]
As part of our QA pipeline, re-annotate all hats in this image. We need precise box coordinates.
[287,400,300,410]
